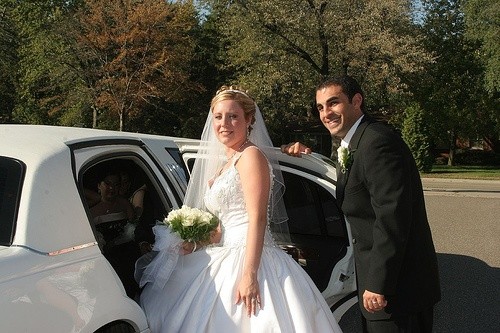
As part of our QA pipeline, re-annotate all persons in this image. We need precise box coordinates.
[88,172,137,268]
[140,85,342,333]
[280,75,440,333]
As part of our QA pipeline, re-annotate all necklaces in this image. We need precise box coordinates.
[217,139,250,176]
[99,197,116,214]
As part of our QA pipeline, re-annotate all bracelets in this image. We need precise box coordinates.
[191,241,197,253]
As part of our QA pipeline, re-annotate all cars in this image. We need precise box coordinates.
[0,124,363,333]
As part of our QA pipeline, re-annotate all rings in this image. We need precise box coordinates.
[372,302,378,304]
[251,298,256,301]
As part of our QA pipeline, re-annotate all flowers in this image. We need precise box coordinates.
[163,205,219,252]
[337,146,352,174]
